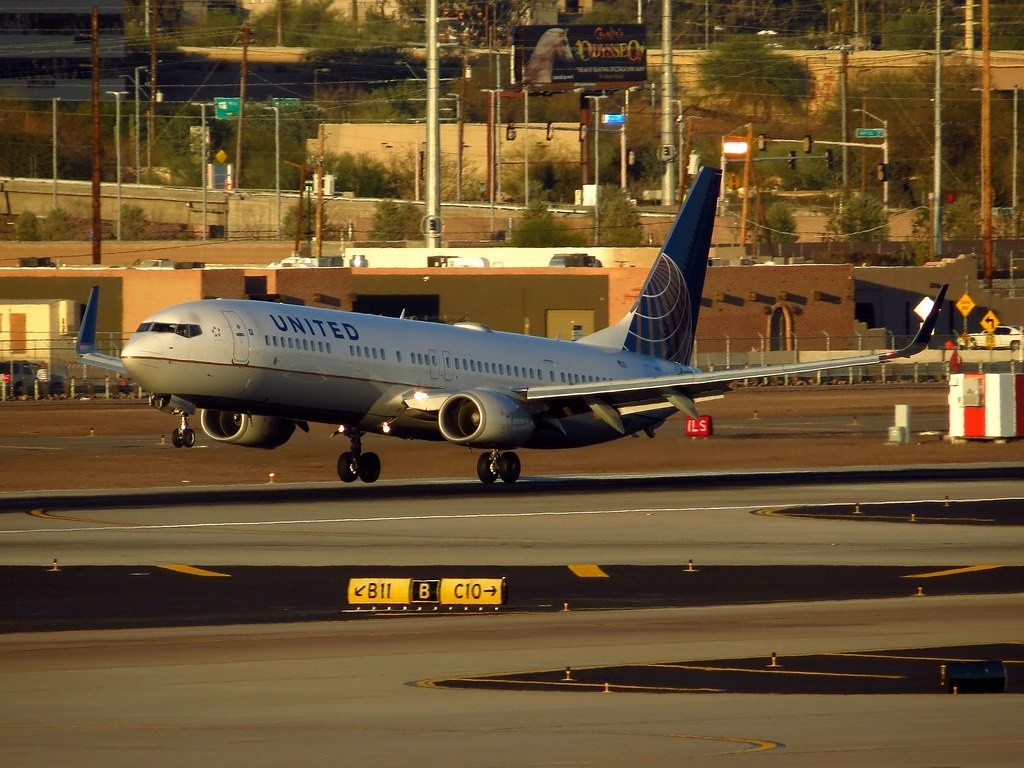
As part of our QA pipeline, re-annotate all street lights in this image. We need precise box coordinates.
[105,90,129,241]
[853,108,891,209]
[971,87,995,237]
[192,102,216,242]
[51,97,62,209]
[672,99,683,186]
[481,88,505,233]
[134,65,148,186]
[625,86,641,119]
[1010,83,1020,239]
[264,107,282,241]
[585,95,609,246]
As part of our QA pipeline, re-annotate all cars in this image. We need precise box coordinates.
[956,326,1024,349]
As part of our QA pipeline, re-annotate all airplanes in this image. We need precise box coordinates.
[75,166,950,483]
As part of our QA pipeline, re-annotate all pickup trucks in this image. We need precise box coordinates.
[0,360,64,397]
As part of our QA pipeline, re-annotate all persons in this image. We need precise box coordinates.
[36,363,52,400]
